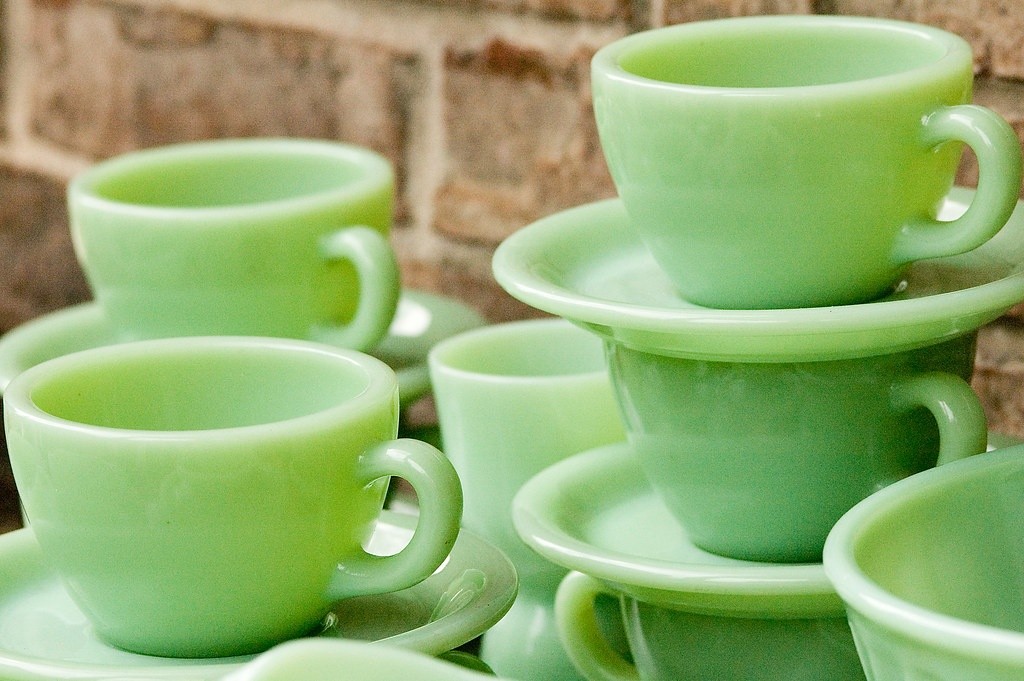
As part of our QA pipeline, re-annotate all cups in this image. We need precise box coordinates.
[553,570,872,681]
[480,579,638,681]
[65,138,402,367]
[589,13,1022,310]
[424,319,637,569]
[2,334,464,658]
[823,442,1024,681]
[602,326,989,565]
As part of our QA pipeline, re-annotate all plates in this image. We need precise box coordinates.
[489,198,1024,364]
[0,504,521,681]
[511,444,848,618]
[0,288,483,410]
